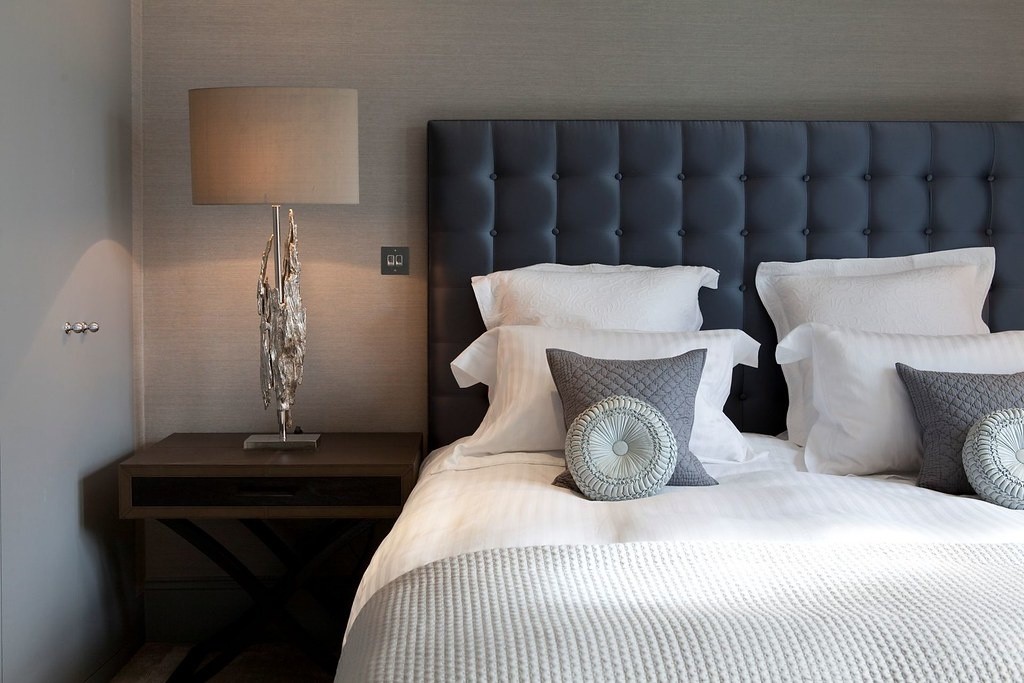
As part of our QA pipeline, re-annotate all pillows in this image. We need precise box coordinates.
[895,362,1024,495]
[470,264,719,332]
[429,325,767,476]
[775,322,1024,476]
[960,408,1024,510]
[755,247,996,447]
[564,395,679,502]
[544,348,719,492]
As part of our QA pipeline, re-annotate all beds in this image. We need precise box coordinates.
[333,117,1024,683]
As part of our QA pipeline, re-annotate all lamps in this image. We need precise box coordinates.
[188,86,361,451]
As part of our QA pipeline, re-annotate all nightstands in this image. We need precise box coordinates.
[118,432,425,643]
